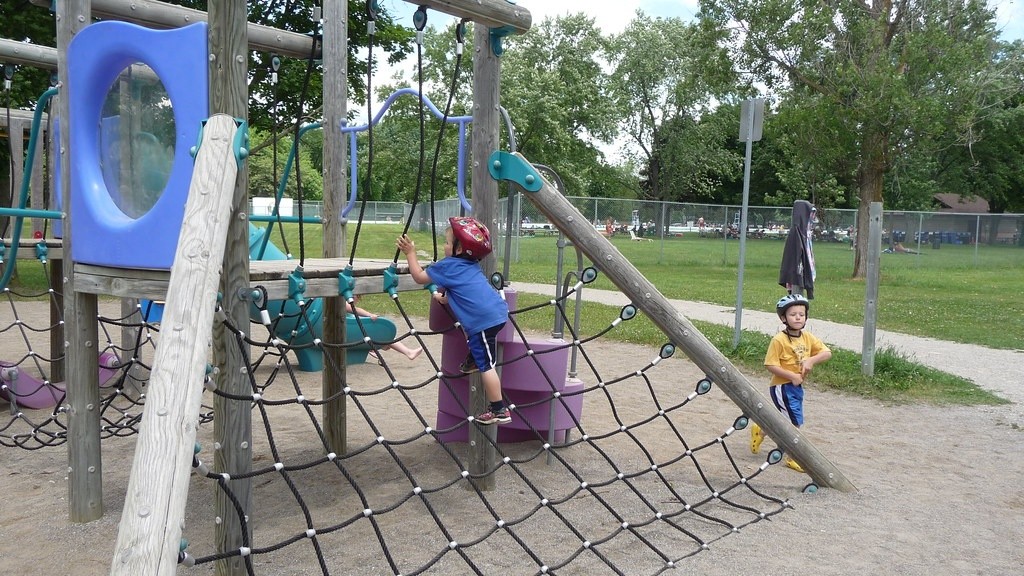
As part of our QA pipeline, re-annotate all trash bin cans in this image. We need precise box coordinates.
[914,232,971,244]
[933,242,940,249]
[648,227,654,235]
[893,231,906,242]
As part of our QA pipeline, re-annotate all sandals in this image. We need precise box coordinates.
[785,457,805,472]
[750,423,764,454]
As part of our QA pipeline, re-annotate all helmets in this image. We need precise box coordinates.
[776,294,809,317]
[449,216,493,260]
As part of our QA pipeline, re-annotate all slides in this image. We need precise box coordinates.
[216,218,397,351]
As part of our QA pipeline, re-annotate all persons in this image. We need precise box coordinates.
[345,295,423,360]
[696,216,927,255]
[606,216,653,240]
[749,294,832,474]
[395,216,512,426]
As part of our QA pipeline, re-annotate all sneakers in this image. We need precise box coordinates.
[475,406,512,425]
[461,353,479,374]
